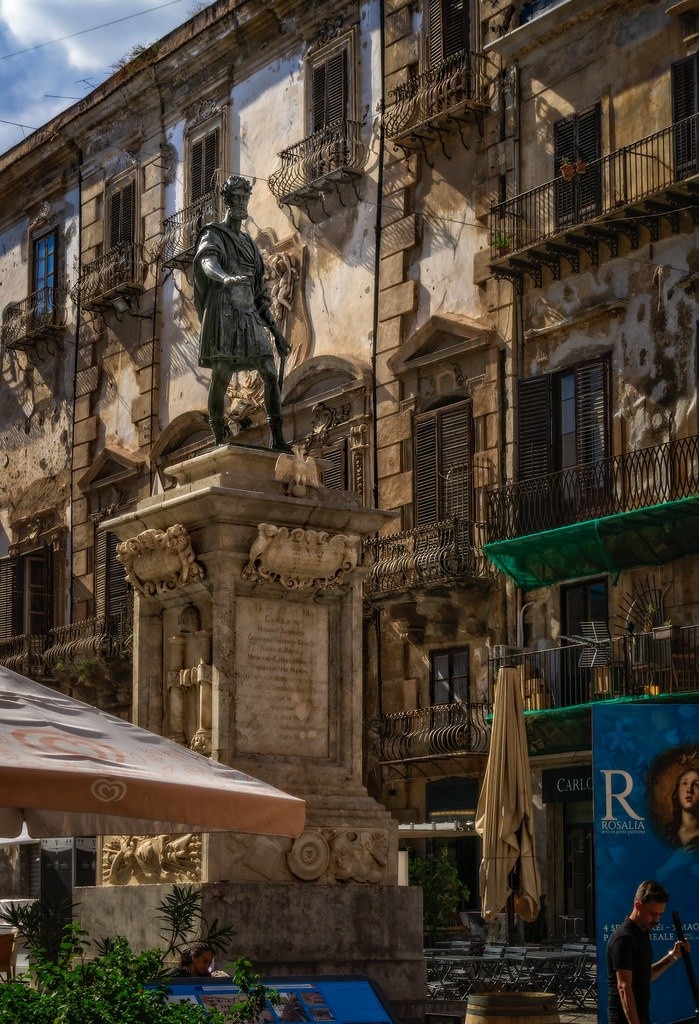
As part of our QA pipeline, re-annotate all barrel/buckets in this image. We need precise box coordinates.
[465,992,561,1024]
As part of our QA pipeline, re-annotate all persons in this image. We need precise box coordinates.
[158,942,215,982]
[606,879,693,1024]
[655,767,699,884]
[194,174,291,452]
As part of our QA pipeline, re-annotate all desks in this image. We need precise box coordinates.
[430,956,520,1000]
[423,948,450,957]
[505,951,590,1009]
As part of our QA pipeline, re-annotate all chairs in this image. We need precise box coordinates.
[558,944,598,1000]
[488,948,529,992]
[424,957,460,1001]
[0,933,14,984]
[557,946,598,1009]
[440,937,473,977]
[10,926,22,983]
[473,947,507,992]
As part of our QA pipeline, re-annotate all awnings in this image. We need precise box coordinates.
[0,665,306,837]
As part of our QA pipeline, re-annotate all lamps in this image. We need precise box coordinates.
[110,296,152,319]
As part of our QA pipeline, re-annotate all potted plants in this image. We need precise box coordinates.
[652,620,676,640]
[559,158,574,181]
[643,604,656,632]
[489,234,512,257]
[575,158,587,174]
